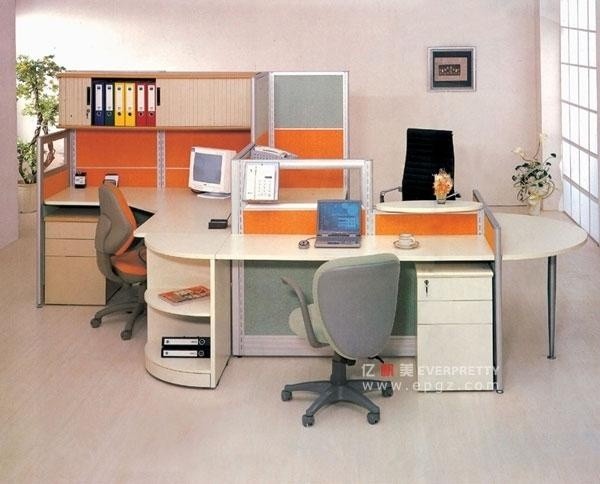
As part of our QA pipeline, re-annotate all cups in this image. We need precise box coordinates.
[103,179,116,186]
[399,233,416,246]
[105,174,119,187]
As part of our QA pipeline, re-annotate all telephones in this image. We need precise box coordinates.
[242,162,279,201]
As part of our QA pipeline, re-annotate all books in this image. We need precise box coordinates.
[158,285,210,307]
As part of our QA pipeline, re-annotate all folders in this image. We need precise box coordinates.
[92,84,156,128]
[161,336,209,358]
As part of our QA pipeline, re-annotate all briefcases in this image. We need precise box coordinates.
[250,144,298,160]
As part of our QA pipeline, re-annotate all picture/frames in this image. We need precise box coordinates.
[426,47,477,91]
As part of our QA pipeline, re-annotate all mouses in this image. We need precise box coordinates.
[298,240,308,248]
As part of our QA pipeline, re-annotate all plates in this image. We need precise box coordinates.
[393,240,420,249]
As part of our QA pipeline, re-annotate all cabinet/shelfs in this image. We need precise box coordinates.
[142,235,230,389]
[57,72,253,132]
[42,218,120,306]
[413,263,495,393]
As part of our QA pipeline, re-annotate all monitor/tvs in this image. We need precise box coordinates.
[187,146,237,200]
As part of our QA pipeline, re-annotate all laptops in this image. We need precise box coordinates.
[313,200,362,248]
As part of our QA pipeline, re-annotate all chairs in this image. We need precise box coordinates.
[279,252,401,427]
[91,187,148,339]
[380,128,461,202]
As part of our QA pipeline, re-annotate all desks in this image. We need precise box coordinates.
[217,232,499,394]
[42,185,233,238]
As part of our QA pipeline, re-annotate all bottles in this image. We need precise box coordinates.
[74,171,87,188]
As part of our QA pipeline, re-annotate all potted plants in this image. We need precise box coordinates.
[14,53,62,214]
